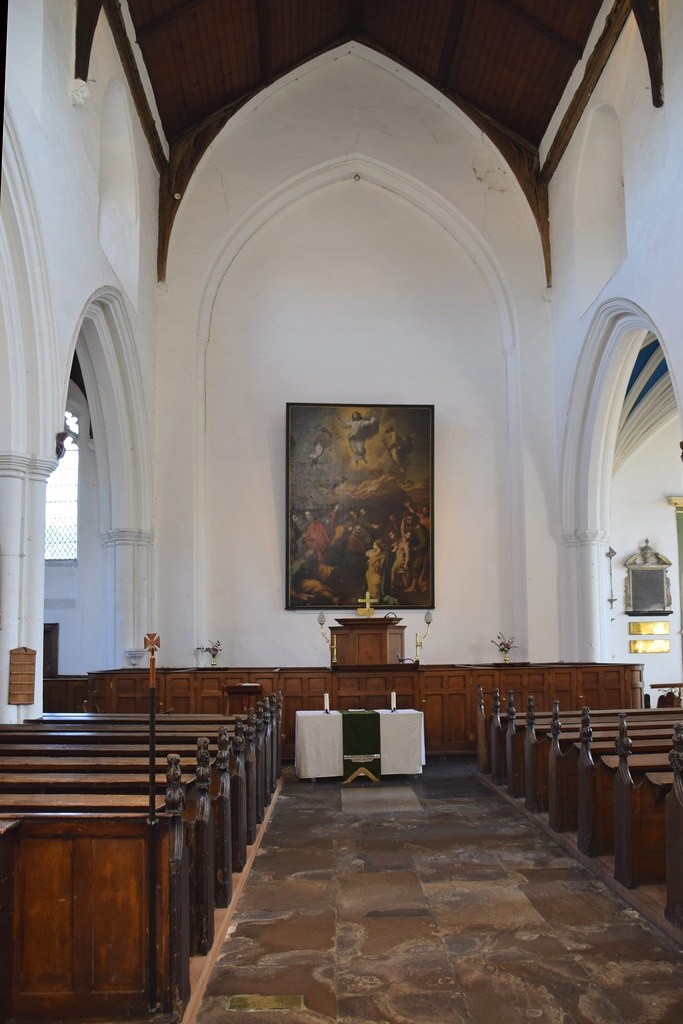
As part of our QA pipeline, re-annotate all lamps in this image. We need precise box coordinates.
[416,611,433,665]
[318,611,338,667]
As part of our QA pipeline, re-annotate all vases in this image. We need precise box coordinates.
[212,658,217,667]
[504,653,510,663]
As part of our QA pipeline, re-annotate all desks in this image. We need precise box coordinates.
[477,685,683,931]
[295,709,426,784]
[0,690,282,1024]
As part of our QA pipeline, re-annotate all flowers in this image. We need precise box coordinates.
[491,631,520,654]
[206,640,222,658]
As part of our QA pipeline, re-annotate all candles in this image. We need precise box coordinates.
[391,690,396,709]
[324,692,329,710]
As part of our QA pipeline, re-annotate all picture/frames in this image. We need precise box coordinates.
[286,403,435,610]
